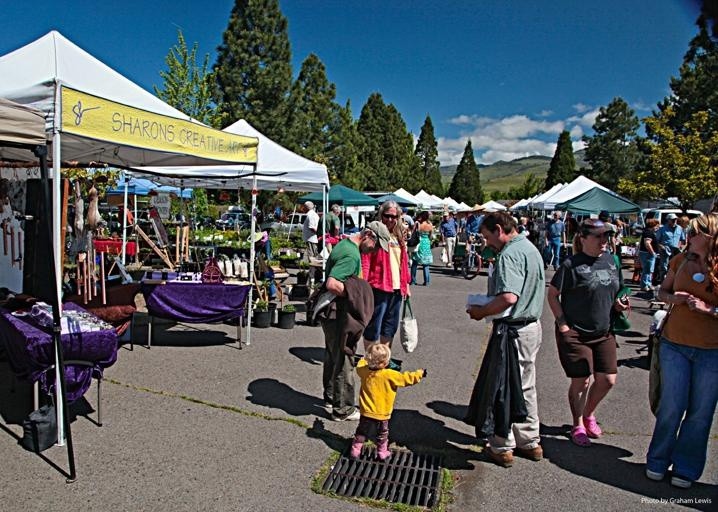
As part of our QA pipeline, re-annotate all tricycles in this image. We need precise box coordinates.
[452,232,496,280]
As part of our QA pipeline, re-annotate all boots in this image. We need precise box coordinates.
[376,440,392,460]
[349,436,364,461]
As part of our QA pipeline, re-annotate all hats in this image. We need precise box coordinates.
[470,203,486,212]
[443,211,450,216]
[664,213,678,220]
[364,221,391,253]
[575,218,614,240]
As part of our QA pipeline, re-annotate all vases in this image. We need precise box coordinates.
[269,302,276,326]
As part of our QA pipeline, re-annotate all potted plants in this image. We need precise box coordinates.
[277,304,299,329]
[252,300,272,329]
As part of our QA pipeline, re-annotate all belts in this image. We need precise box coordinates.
[495,317,540,328]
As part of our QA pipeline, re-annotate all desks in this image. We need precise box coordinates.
[87,279,252,349]
[63,283,139,352]
[1,288,112,425]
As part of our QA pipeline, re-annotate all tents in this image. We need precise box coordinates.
[0,29,260,446]
[394,187,508,213]
[117,117,329,344]
[509,176,642,214]
[1,95,80,485]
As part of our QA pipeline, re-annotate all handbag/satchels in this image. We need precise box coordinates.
[647,252,692,417]
[613,255,636,332]
[201,266,225,285]
[406,223,421,249]
[398,293,420,354]
[438,243,449,265]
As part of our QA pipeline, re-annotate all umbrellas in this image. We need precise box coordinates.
[299,185,378,234]
[377,193,417,209]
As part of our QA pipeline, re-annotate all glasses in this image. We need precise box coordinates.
[381,212,400,220]
[687,229,708,239]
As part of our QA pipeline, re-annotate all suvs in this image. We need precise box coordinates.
[260,214,307,234]
[642,208,704,229]
[216,213,252,229]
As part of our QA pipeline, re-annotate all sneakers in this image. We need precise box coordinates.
[325,401,334,415]
[670,473,693,489]
[481,446,514,467]
[331,408,361,423]
[584,414,604,438]
[384,357,402,373]
[515,445,544,461]
[568,425,590,446]
[646,469,665,480]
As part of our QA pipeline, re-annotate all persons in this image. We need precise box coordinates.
[439,211,459,267]
[409,211,433,286]
[638,219,659,292]
[518,217,529,237]
[464,204,487,256]
[466,214,545,466]
[598,210,616,256]
[349,344,426,462]
[656,214,686,280]
[401,207,414,228]
[547,218,631,448]
[317,204,341,253]
[320,220,390,422]
[362,200,411,355]
[545,211,566,271]
[645,214,718,488]
[303,201,320,261]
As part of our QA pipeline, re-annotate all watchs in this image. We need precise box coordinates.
[713,306,718,317]
